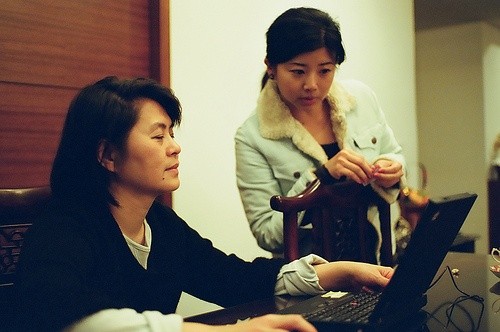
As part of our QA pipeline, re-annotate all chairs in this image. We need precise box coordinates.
[271,179,393,268]
[0,188,55,330]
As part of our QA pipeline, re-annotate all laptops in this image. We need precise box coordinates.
[275,192,478,332]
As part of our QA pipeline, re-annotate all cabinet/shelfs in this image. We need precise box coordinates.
[449,234,478,254]
[488,180,500,254]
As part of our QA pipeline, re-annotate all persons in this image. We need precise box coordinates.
[234,6,407,258]
[0,74,395,332]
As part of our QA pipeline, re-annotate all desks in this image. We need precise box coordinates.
[183,250,500,332]
